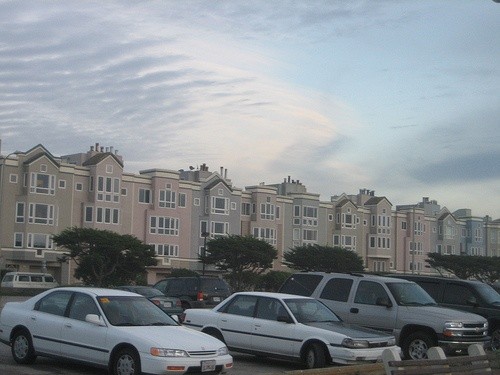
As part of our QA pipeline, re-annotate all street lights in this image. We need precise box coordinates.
[201,232,210,275]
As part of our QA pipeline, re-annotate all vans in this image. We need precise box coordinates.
[1,272,61,289]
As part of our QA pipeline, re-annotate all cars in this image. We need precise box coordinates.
[179,290,401,369]
[98,286,184,317]
[0,286,234,375]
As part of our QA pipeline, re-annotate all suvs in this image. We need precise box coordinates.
[270,267,489,360]
[154,277,233,309]
[376,273,500,351]
